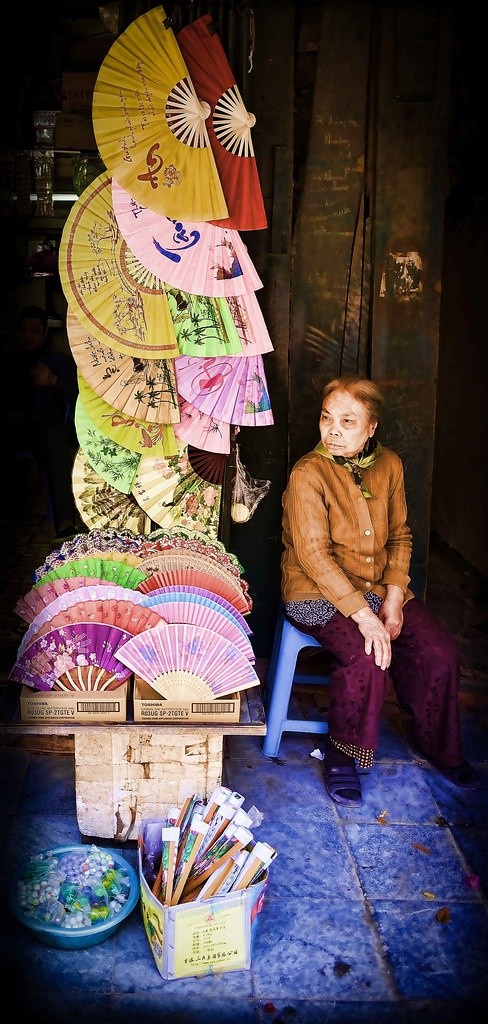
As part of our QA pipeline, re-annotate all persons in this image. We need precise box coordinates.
[279,375,485,808]
[0,306,76,539]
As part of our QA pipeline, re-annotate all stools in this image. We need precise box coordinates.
[260,610,330,758]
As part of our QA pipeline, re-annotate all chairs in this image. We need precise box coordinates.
[1,400,71,523]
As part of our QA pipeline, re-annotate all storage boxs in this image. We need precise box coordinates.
[74,733,223,840]
[137,825,270,980]
[21,664,242,725]
[33,41,108,151]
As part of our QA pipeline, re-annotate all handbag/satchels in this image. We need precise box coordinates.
[230,440,273,525]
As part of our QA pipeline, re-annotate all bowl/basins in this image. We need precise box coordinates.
[16,845,140,950]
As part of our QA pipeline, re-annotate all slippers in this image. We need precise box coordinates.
[420,745,484,792]
[323,764,366,808]
